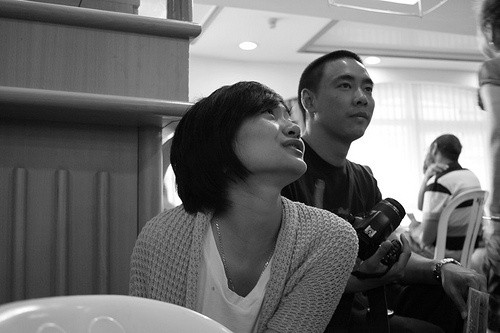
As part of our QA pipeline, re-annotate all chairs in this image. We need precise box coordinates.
[434,189,488,268]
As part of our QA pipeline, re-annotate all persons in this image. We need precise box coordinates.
[282,51,487,333]
[476,0,500,299]
[398,134,482,262]
[128,81,359,333]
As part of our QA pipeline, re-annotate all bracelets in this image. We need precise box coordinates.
[489,213,500,222]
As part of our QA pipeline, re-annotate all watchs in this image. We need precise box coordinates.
[432,258,463,283]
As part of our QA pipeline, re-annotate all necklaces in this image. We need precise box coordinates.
[215,217,276,297]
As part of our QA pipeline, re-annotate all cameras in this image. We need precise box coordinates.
[344,197,407,267]
